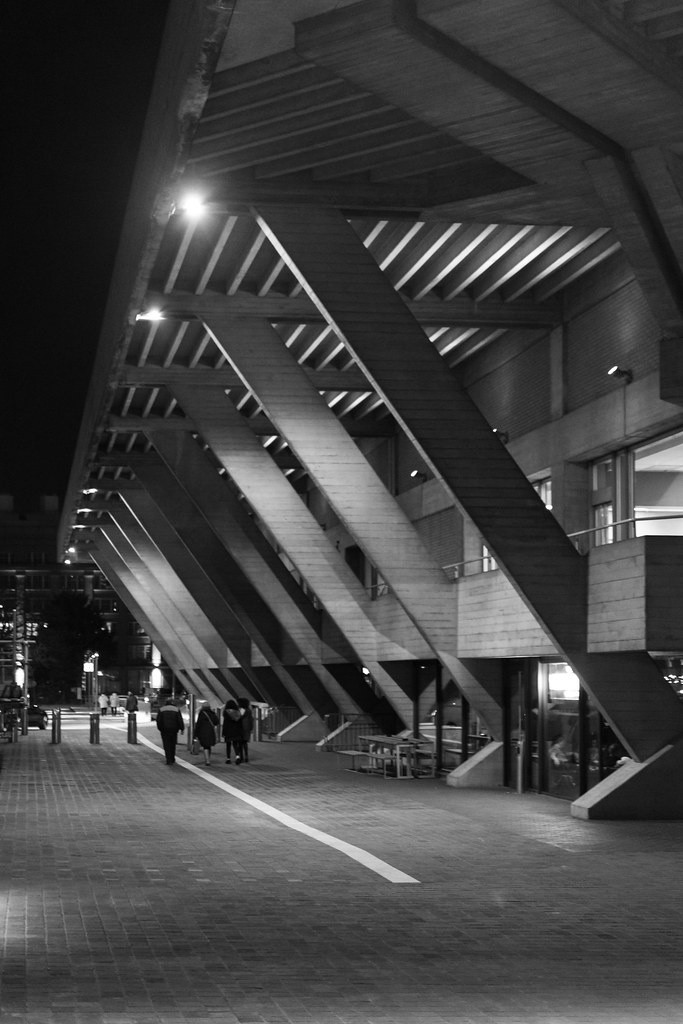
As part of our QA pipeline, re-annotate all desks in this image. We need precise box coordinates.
[358,734,436,780]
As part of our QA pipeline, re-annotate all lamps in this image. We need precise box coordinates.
[411,470,425,479]
[607,366,632,379]
[493,428,508,441]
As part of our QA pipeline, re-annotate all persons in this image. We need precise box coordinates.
[222,698,254,766]
[194,702,220,765]
[157,698,184,765]
[98,692,119,716]
[81,688,86,703]
[141,686,146,696]
[126,692,138,714]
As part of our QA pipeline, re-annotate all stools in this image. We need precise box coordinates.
[414,749,441,777]
[446,748,475,756]
[337,750,398,780]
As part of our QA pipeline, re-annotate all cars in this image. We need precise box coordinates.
[0,698,48,730]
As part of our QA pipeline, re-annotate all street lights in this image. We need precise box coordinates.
[88,652,99,713]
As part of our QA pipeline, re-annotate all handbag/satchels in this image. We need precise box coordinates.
[135,706,139,711]
[190,736,201,755]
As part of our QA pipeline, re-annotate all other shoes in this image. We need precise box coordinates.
[206,762,211,766]
[226,759,231,764]
[235,756,241,765]
